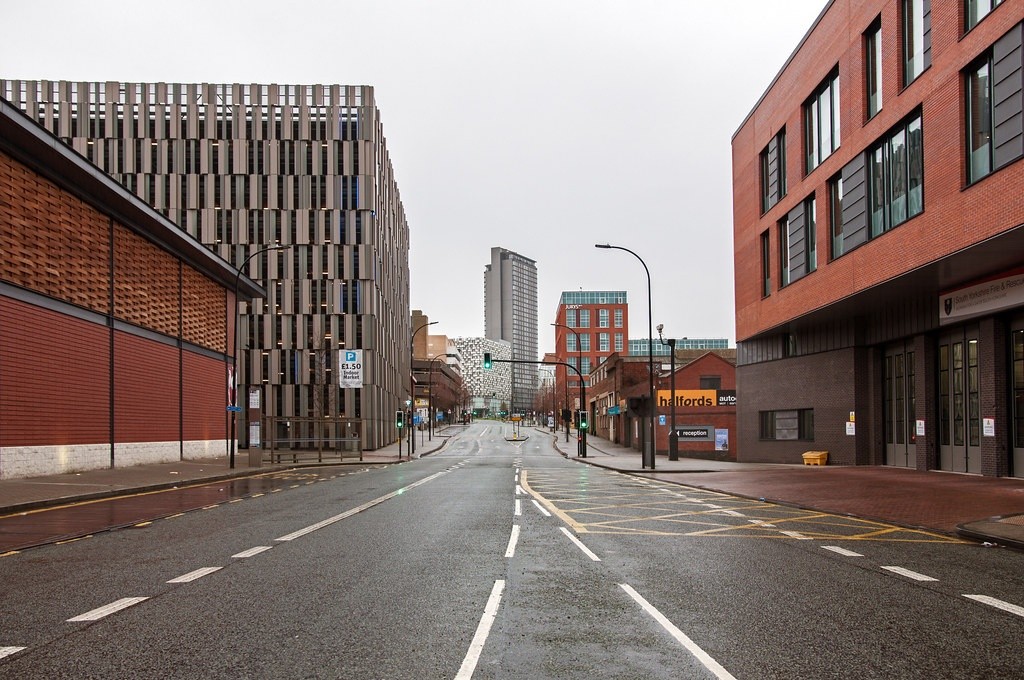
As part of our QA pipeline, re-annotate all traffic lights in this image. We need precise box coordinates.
[395,411,404,429]
[579,411,588,429]
[473,411,477,415]
[484,353,492,369]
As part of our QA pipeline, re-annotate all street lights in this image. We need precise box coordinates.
[539,369,555,433]
[410,322,440,451]
[595,243,655,469]
[229,245,290,468]
[545,354,569,442]
[550,323,582,457]
[428,353,456,440]
